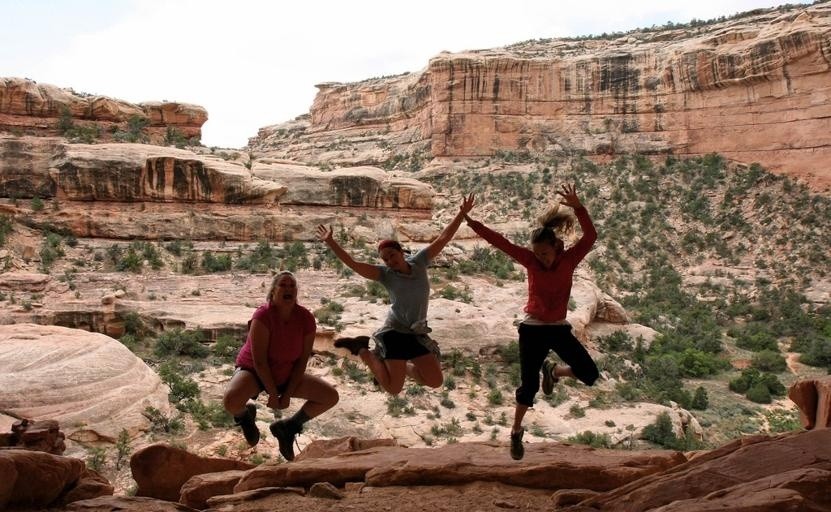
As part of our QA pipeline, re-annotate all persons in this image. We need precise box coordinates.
[223,269,339,462]
[459,183,600,460]
[315,192,475,396]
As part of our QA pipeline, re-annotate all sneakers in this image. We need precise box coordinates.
[541,357,559,395]
[234,403,261,448]
[270,417,296,462]
[509,426,525,461]
[332,335,369,355]
